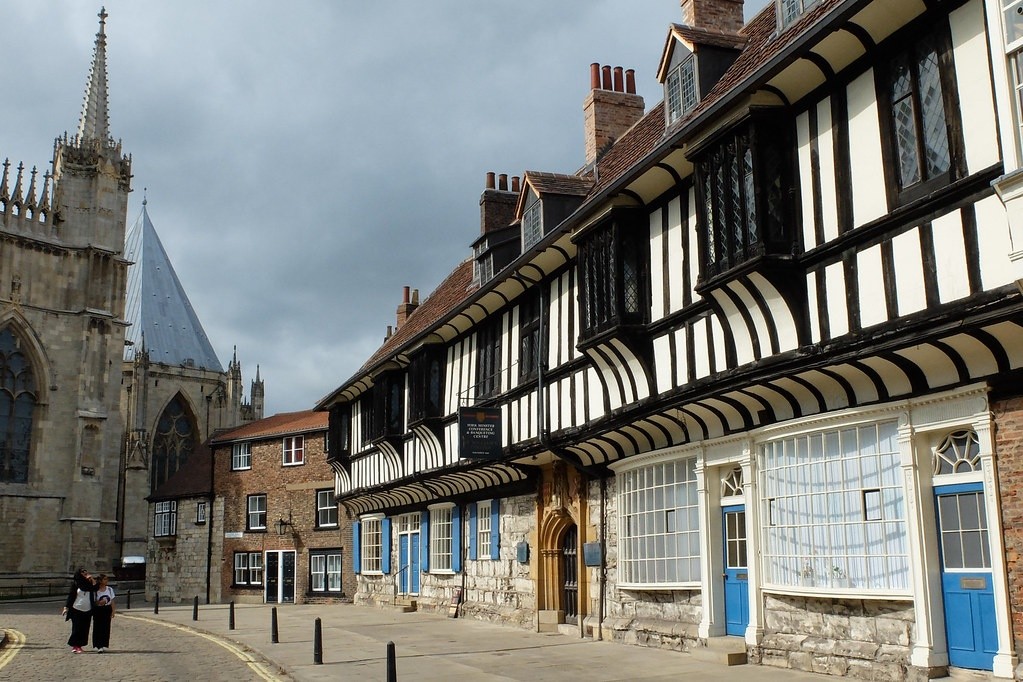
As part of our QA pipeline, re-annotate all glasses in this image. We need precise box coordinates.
[80,569,87,573]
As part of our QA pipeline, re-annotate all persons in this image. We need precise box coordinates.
[63,567,98,653]
[92,574,115,654]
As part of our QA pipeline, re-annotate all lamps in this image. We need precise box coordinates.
[273,512,299,538]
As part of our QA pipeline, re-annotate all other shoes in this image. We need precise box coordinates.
[71,649,82,654]
[97,648,104,653]
